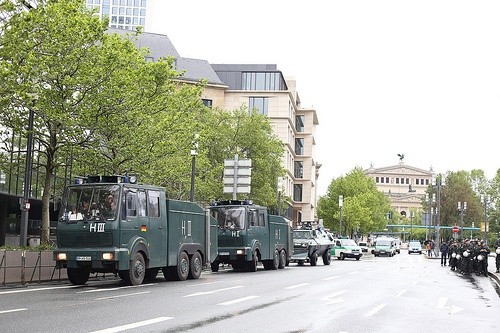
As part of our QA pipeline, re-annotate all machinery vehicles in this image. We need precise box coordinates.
[290,227,336,267]
[52,174,219,288]
[204,200,295,271]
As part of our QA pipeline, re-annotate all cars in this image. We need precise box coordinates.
[326,236,422,261]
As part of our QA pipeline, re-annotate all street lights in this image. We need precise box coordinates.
[429,206,438,244]
[408,211,416,241]
[424,191,436,252]
[189,134,200,201]
[431,172,446,256]
[457,200,468,241]
[480,193,492,246]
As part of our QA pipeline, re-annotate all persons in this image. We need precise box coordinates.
[425,236,500,277]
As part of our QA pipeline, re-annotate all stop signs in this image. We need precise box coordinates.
[452,227,459,233]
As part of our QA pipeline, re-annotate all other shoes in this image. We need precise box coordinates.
[436,262,490,282]
